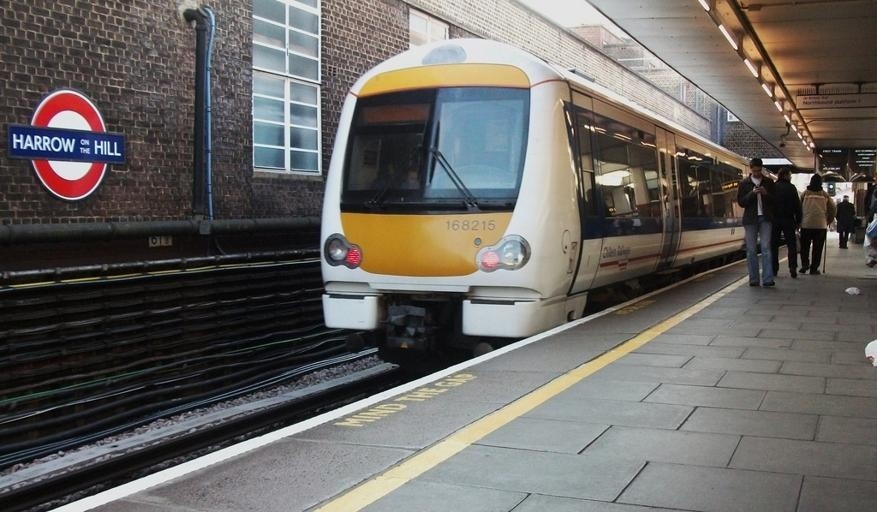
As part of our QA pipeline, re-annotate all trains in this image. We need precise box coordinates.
[319,37,792,369]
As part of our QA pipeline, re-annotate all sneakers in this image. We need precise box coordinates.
[773,271,777,276]
[790,266,821,278]
[749,282,775,287]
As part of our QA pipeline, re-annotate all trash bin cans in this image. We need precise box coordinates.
[851,216,866,242]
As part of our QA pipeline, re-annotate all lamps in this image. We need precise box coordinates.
[697,0,811,152]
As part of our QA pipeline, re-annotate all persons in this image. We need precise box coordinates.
[735,158,777,287]
[833,195,856,249]
[766,164,802,279]
[797,173,835,274]
